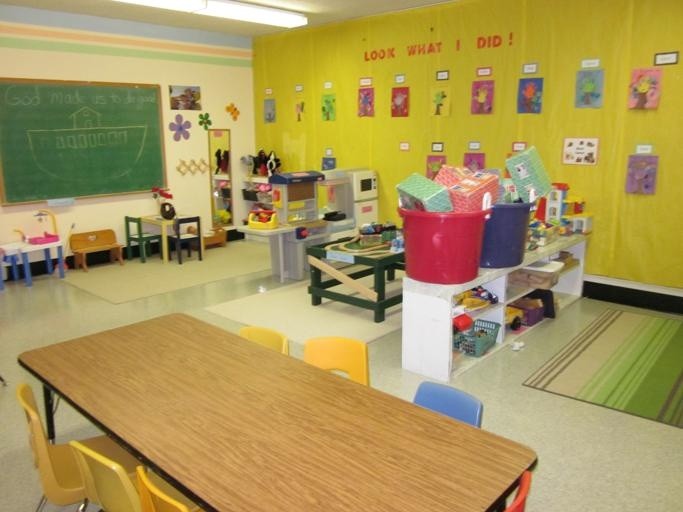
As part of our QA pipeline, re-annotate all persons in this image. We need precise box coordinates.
[171,87,197,110]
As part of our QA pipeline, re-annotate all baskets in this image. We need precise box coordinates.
[508,302,544,326]
[458,319,501,358]
[500,178,519,200]
[433,165,464,186]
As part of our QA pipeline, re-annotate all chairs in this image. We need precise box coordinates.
[237,326,289,356]
[132,464,203,511]
[409,380,483,430]
[66,438,144,512]
[13,384,143,511]
[300,336,370,387]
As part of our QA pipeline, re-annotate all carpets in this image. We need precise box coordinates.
[522,307,682,427]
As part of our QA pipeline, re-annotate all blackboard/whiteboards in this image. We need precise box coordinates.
[0,78,169,207]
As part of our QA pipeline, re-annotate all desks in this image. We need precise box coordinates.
[0,235,66,292]
[16,311,537,511]
[301,224,407,323]
[140,214,205,267]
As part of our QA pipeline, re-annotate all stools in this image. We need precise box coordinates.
[121,216,162,264]
[168,216,204,266]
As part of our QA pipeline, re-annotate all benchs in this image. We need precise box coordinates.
[68,228,125,274]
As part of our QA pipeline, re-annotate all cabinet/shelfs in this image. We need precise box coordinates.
[401,231,595,382]
[236,210,357,283]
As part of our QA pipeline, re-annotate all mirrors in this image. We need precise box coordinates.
[206,127,234,231]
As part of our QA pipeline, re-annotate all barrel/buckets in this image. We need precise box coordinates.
[392,203,496,287]
[481,198,532,268]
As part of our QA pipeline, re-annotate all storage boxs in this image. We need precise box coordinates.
[508,262,560,290]
[431,163,474,188]
[506,298,544,327]
